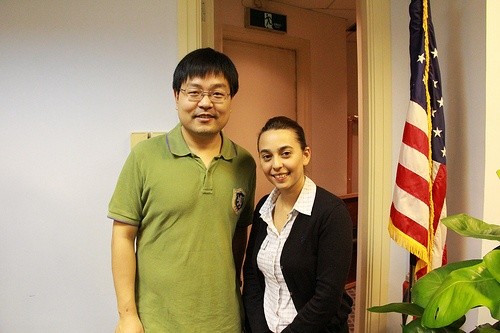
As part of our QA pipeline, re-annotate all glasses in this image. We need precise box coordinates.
[179,87,231,104]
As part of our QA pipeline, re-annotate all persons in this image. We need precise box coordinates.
[241,116,354,332]
[106,47,257,333]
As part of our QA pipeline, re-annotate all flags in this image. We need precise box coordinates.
[387,0,447,325]
